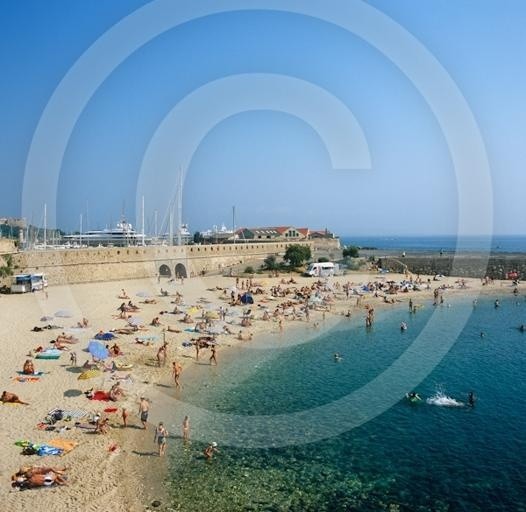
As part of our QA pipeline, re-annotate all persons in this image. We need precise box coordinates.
[255,261,525,336]
[405,391,421,404]
[402,250,407,258]
[1,266,255,491]
[440,247,444,257]
[468,392,477,402]
[333,353,343,361]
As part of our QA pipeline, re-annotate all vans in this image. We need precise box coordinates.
[305,262,336,279]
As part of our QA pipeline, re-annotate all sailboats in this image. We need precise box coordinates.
[19,172,251,251]
